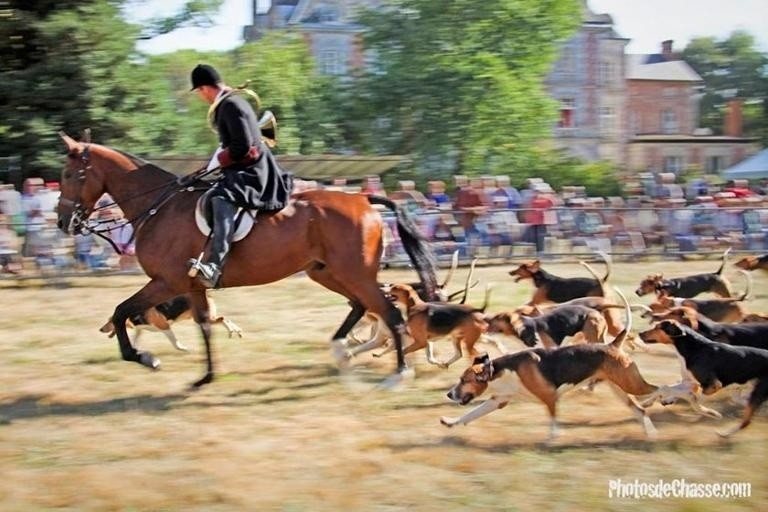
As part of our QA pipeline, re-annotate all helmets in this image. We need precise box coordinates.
[189,64,224,93]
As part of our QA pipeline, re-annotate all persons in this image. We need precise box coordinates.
[172,61,269,287]
[2,169,768,275]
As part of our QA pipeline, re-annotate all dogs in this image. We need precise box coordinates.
[344,250,506,370]
[440,250,768,438]
[99,287,242,351]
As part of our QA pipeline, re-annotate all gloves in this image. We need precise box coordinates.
[217,148,233,167]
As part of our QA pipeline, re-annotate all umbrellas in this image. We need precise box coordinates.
[718,148,768,180]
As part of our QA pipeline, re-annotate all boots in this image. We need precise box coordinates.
[187,195,239,290]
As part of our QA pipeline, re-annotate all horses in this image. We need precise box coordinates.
[55,128,440,388]
